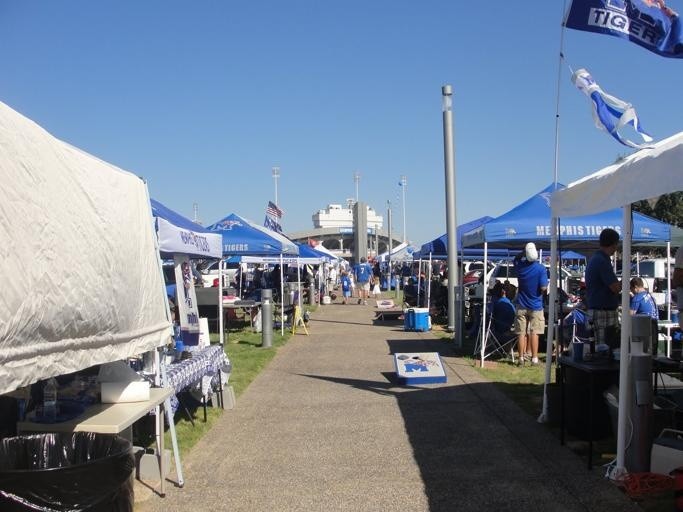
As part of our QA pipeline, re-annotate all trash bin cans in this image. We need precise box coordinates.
[1,432,134,512]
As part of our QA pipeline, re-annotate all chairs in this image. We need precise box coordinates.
[474,301,595,363]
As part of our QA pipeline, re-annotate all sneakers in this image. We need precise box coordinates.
[358,299,361,304]
[363,302,367,305]
[531,360,542,366]
[517,360,525,367]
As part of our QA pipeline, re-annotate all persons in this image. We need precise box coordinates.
[233,256,518,356]
[672,242,683,331]
[629,277,659,319]
[512,243,547,365]
[586,228,622,354]
[540,277,586,351]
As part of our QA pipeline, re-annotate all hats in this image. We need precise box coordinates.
[525,242,538,262]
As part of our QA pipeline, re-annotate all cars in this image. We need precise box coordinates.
[162,258,264,305]
[463,256,676,307]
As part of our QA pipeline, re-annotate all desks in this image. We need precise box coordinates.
[557,358,621,471]
[17,342,225,498]
[223,302,262,328]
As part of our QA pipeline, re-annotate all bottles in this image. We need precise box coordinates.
[44,379,57,421]
[589,336,595,354]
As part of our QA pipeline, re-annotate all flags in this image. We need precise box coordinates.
[266,201,284,218]
[264,215,282,234]
[563,0,683,59]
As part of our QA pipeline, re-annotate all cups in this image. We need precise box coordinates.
[573,342,584,363]
[176,341,184,353]
